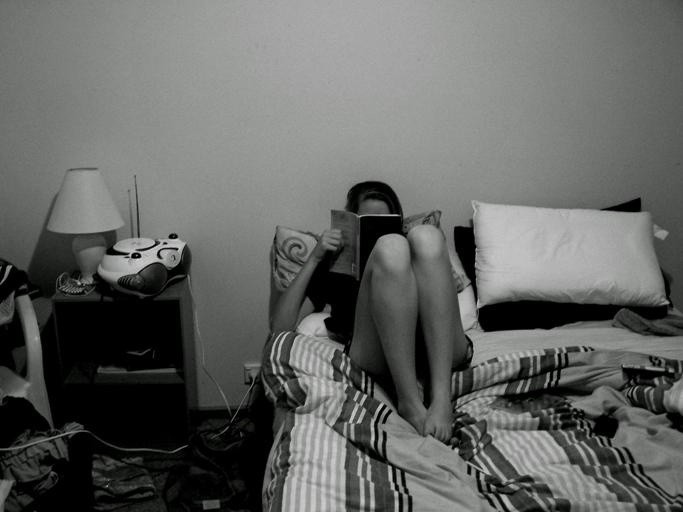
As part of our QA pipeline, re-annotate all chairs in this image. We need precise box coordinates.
[0,258,55,432]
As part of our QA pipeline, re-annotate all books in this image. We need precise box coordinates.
[327,209,402,281]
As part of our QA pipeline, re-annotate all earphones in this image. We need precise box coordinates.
[81,279,86,286]
[74,280,81,286]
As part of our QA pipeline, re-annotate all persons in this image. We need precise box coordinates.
[269,181,474,442]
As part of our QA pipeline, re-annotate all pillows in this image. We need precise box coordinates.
[275,208,478,332]
[454,197,670,333]
[470,200,671,311]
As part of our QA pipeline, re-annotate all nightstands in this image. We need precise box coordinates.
[52,278,197,435]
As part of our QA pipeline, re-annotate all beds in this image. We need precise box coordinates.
[271,197,683,512]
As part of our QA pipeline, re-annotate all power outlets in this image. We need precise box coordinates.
[244,364,261,384]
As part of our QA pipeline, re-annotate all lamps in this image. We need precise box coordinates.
[45,167,125,287]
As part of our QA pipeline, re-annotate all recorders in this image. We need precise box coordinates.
[93,233,191,298]
